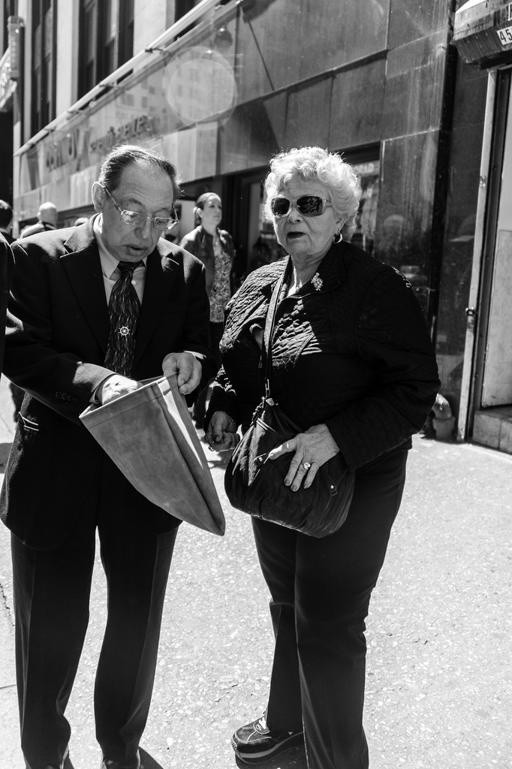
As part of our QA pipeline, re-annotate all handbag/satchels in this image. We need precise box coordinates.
[81,376,228,541]
[223,401,352,542]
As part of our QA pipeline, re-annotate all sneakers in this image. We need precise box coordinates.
[230,707,304,764]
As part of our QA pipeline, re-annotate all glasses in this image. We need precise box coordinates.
[104,183,179,233]
[265,194,328,221]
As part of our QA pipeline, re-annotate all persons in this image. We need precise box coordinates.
[205,146,440,769]
[0,142,214,769]
[179,188,238,427]
[0,197,89,427]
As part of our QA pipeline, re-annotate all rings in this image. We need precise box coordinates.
[301,463,311,470]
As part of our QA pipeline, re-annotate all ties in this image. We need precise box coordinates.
[99,257,144,376]
